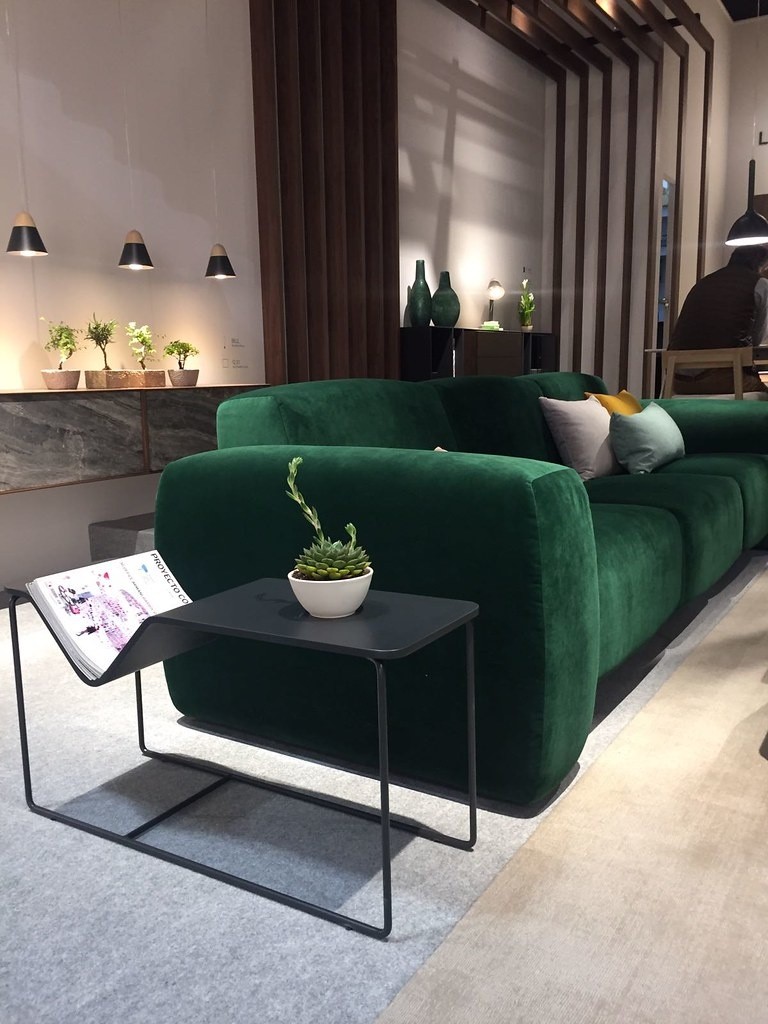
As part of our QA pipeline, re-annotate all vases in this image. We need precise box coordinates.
[431,271,461,328]
[125,368,167,388]
[409,260,431,327]
[40,369,81,390]
[520,325,533,332]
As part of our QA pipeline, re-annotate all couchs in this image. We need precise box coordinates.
[153,372,768,810]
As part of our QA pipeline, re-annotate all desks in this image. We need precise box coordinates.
[400,326,557,382]
[643,344,768,399]
[0,383,272,496]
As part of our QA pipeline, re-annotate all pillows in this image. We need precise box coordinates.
[538,394,618,482]
[610,400,686,474]
[584,390,643,415]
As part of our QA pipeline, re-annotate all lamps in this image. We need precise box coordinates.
[203,0,238,280]
[3,0,50,258]
[116,0,156,272]
[723,0,768,247]
[480,280,506,331]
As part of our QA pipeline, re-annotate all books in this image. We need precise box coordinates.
[25,550,194,681]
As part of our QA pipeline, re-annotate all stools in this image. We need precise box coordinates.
[87,512,154,563]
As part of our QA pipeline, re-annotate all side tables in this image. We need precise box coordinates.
[0,556,479,938]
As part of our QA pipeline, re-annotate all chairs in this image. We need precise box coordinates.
[662,346,768,401]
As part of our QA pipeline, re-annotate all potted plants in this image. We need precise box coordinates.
[83,311,127,389]
[162,339,201,387]
[284,457,374,619]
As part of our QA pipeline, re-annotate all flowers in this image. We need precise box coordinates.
[517,277,536,326]
[39,316,88,370]
[125,320,166,370]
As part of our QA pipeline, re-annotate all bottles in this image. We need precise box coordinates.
[409,259,432,327]
[431,271,460,328]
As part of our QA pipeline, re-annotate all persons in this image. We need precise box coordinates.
[667,246,768,393]
